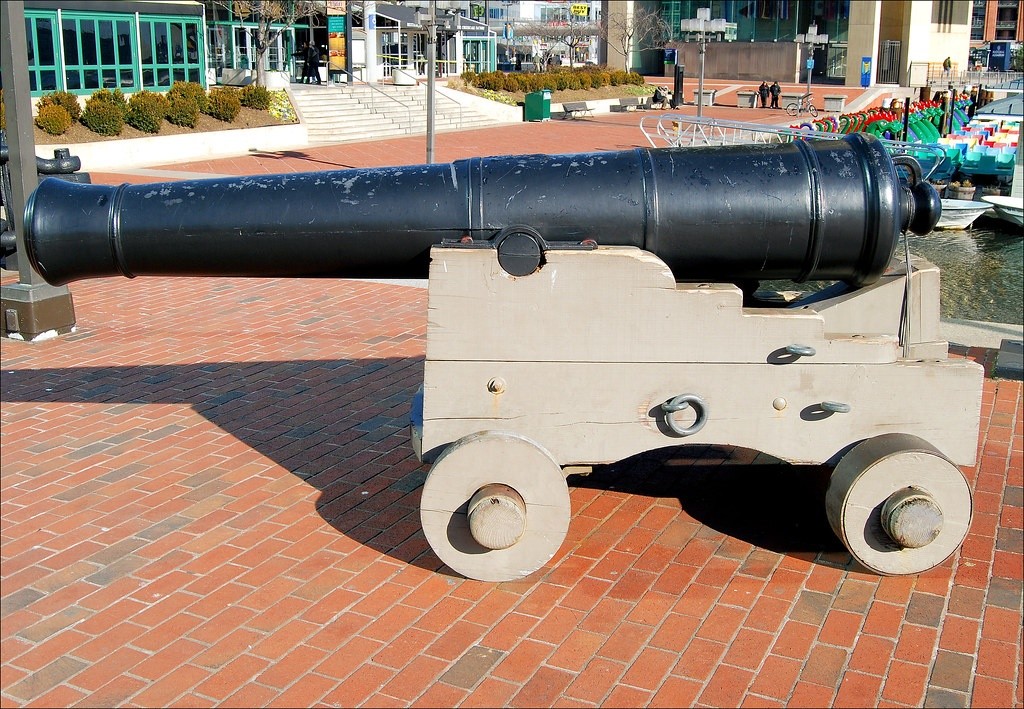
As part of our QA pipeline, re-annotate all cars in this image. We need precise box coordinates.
[969,39,1024,72]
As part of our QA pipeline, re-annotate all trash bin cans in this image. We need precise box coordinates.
[988,41,1011,72]
[525,90,552,121]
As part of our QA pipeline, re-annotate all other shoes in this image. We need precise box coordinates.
[770,106,773,109]
[775,107,778,109]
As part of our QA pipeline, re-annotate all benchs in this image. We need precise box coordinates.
[562,102,595,121]
[648,97,661,109]
[619,98,647,113]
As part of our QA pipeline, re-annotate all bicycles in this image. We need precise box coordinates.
[785,89,818,118]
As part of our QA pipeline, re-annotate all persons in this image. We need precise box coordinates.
[653,85,680,111]
[758,80,770,108]
[769,80,781,108]
[943,56,951,76]
[291,40,321,84]
